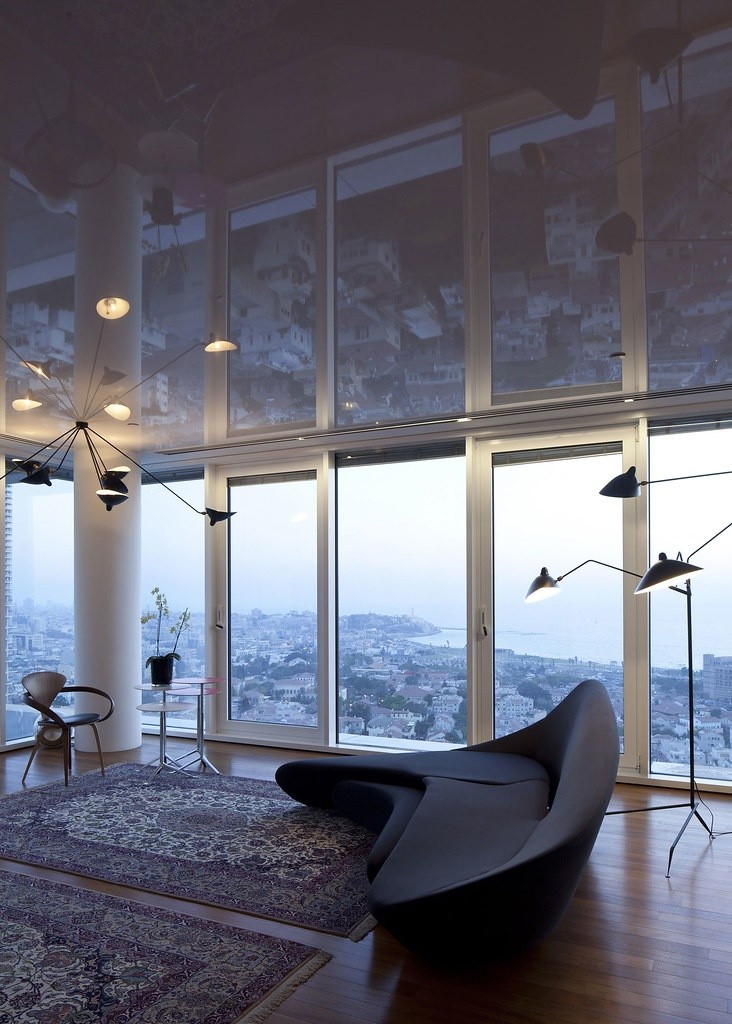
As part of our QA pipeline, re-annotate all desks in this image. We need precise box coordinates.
[135,677,228,785]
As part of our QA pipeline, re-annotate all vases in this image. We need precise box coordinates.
[150,657,174,684]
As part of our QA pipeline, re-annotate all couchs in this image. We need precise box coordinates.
[279,679,621,972]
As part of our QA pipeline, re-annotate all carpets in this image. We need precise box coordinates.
[0,761,379,943]
[0,868,336,1024]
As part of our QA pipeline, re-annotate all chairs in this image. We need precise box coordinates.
[20,671,115,788]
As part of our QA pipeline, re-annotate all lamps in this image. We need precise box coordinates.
[0,421,238,526]
[520,0,732,257]
[525,466,732,879]
[0,295,240,422]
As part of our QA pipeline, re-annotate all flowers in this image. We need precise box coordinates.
[141,586,192,667]
